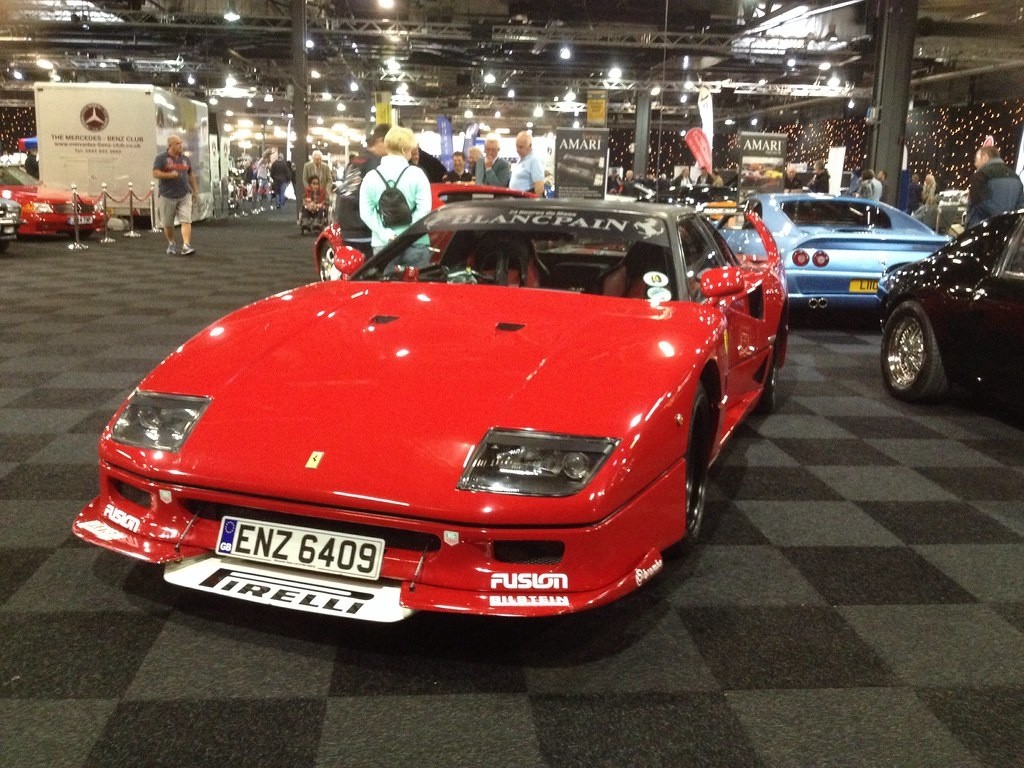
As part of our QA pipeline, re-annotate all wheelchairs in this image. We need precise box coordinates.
[299,199,330,235]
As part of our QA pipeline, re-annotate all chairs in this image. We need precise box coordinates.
[472,229,534,289]
[600,241,680,302]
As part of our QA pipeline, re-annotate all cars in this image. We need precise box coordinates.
[877,208,1024,423]
[0,166,108,240]
[0,197,25,251]
[310,181,537,287]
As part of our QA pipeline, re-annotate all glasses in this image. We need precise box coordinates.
[313,181,319,186]
[485,148,498,152]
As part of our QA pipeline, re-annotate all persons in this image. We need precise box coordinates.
[25,150,39,180]
[302,149,338,227]
[784,161,829,193]
[966,147,1024,231]
[448,131,543,198]
[853,168,937,217]
[334,124,447,272]
[228,145,296,214]
[153,135,198,255]
[607,165,723,197]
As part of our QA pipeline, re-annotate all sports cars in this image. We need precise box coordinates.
[71,197,788,625]
[716,192,954,320]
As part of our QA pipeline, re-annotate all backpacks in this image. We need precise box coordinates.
[375,165,417,229]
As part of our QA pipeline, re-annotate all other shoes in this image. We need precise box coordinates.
[251,206,265,214]
[233,211,248,219]
[270,205,283,210]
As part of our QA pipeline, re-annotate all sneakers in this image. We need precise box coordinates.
[167,241,178,256]
[181,245,195,256]
